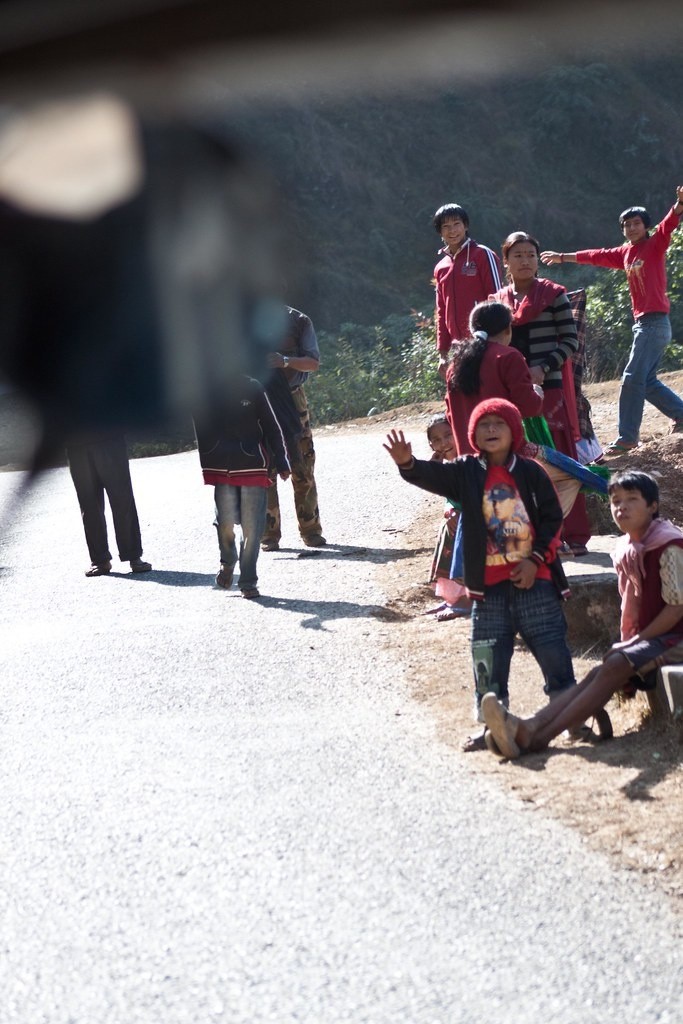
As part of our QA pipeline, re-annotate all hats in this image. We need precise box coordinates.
[467,398,523,453]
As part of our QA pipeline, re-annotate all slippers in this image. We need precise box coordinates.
[670,420,683,433]
[422,603,471,621]
[481,692,521,759]
[605,438,638,455]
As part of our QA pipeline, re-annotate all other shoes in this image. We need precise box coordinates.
[570,542,588,556]
[461,725,488,752]
[216,554,238,588]
[130,561,151,572]
[261,541,279,551]
[303,537,326,546]
[241,588,259,598]
[85,562,112,576]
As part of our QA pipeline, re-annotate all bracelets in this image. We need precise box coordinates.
[528,557,540,567]
[676,199,683,205]
[540,363,550,377]
[398,458,413,468]
[559,253,565,263]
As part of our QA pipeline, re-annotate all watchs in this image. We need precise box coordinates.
[283,356,289,369]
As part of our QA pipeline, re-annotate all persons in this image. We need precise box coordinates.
[67,422,152,577]
[480,469,683,758]
[421,204,593,622]
[539,185,683,456]
[260,305,327,551]
[384,398,586,749]
[190,370,292,601]
